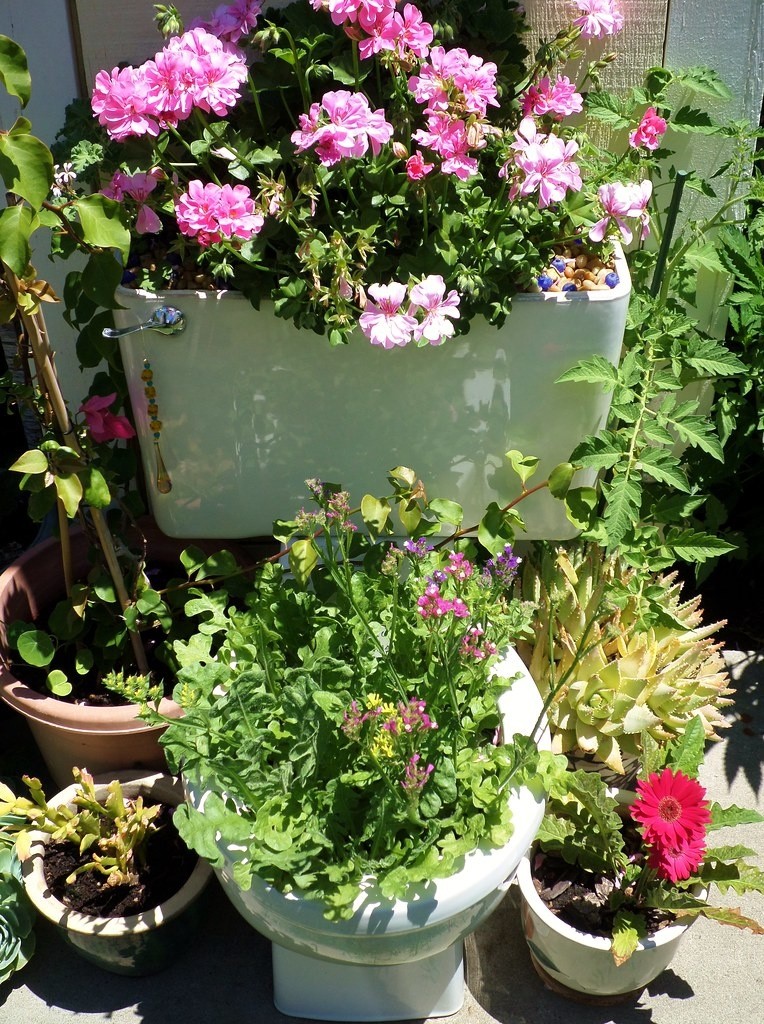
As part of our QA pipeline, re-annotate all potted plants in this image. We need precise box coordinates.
[0,14,597,976]
[515,547,736,791]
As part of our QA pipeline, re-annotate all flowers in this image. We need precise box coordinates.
[49,0,726,447]
[530,716,764,967]
[103,479,519,923]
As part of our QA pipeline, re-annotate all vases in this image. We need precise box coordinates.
[517,848,702,996]
